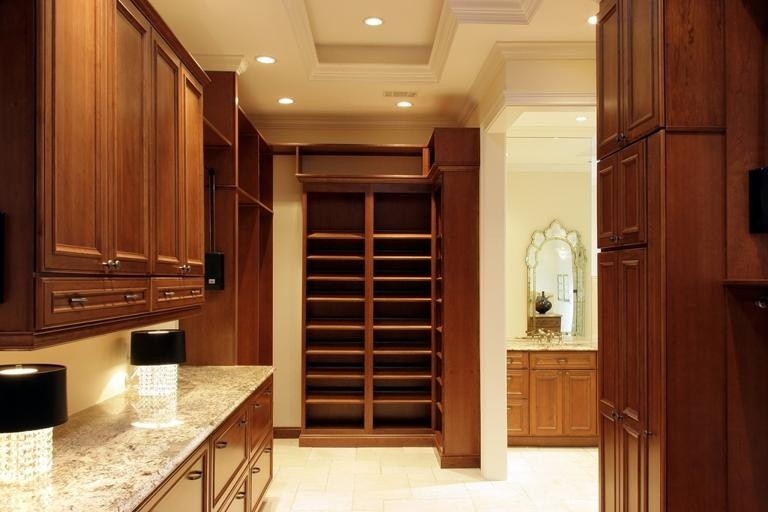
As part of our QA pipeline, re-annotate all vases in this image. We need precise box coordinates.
[536,291,552,314]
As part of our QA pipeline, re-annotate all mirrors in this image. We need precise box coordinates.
[525,219,588,338]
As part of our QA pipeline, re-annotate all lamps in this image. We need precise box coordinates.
[130,329,185,397]
[0,364,67,482]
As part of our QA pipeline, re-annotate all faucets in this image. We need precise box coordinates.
[546,330,554,344]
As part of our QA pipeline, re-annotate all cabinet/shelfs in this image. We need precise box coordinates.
[530,351,598,447]
[527,314,563,336]
[295,127,480,467]
[132,373,273,512]
[507,351,530,446]
[0,1,211,350]
[596,0,768,512]
[179,70,272,364]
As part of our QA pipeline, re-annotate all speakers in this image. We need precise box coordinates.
[204,252,225,291]
[747,167,768,234]
